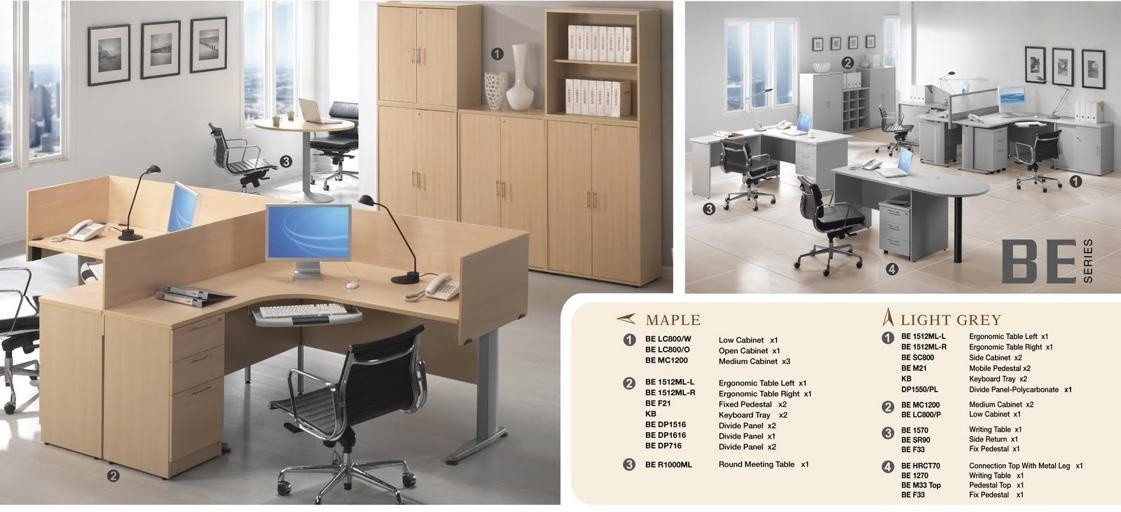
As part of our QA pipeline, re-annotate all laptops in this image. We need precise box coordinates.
[876,147,914,178]
[297,97,343,124]
[782,112,811,137]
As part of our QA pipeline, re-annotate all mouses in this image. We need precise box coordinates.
[1033,114,1039,119]
[346,279,361,292]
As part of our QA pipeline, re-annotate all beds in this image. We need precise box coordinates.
[874,104,914,156]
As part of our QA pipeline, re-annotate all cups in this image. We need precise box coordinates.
[288,111,295,121]
[809,128,818,138]
[273,116,281,126]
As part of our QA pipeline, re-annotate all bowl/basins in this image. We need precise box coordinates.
[813,63,832,73]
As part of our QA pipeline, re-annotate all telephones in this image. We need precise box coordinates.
[861,158,883,171]
[66,219,106,241]
[775,120,792,130]
[968,113,984,123]
[425,274,459,300]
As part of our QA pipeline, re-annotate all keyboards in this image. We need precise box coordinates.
[258,302,350,319]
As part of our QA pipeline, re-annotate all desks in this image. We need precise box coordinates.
[896,84,952,167]
[25,173,288,462]
[952,88,1113,177]
[254,116,355,204]
[689,123,852,199]
[831,156,990,263]
[106,199,529,479]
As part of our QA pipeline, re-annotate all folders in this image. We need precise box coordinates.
[1075,101,1104,123]
[155,285,236,308]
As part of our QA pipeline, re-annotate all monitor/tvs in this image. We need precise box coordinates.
[264,202,353,282]
[961,81,971,94]
[166,180,202,233]
[998,85,1026,118]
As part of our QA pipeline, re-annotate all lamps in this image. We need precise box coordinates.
[118,163,160,240]
[744,88,775,132]
[939,71,956,90]
[356,193,421,285]
[1037,76,1071,121]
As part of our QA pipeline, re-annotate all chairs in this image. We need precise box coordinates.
[309,99,358,190]
[1007,127,1063,195]
[262,325,430,503]
[794,174,865,276]
[717,137,779,211]
[0,262,38,414]
[206,122,279,194]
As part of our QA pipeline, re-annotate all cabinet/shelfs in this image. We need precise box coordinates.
[842,86,866,134]
[961,126,1009,175]
[374,105,458,222]
[856,65,895,130]
[543,6,661,126]
[375,1,483,109]
[543,115,662,287]
[879,193,913,261]
[454,105,546,274]
[798,71,843,135]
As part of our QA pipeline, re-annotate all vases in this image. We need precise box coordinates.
[484,70,508,110]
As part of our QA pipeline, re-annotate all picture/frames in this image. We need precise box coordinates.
[847,35,858,48]
[1051,47,1073,87]
[811,37,823,51]
[1080,48,1105,89]
[140,19,179,80]
[190,16,226,73]
[865,34,875,48]
[86,22,130,87]
[830,37,841,50]
[1024,46,1046,84]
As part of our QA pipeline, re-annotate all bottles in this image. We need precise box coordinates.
[861,55,871,68]
